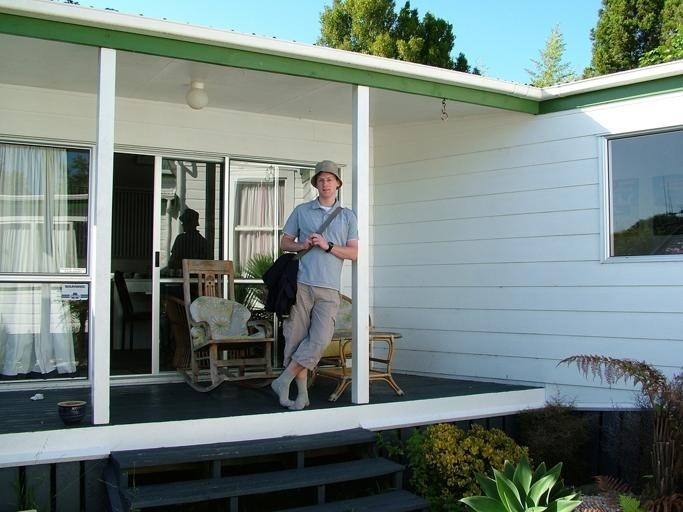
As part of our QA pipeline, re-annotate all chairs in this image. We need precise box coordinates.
[310,292,372,368]
[113,270,151,352]
[175,258,281,393]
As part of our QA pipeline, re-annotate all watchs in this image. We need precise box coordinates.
[325,241,334,253]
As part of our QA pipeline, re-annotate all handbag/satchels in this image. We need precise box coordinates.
[262,253,298,313]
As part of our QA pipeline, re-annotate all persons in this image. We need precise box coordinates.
[162,207,207,352]
[270,159,358,411]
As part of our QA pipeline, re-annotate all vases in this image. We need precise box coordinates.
[57,400,86,425]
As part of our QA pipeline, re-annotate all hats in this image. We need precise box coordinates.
[310,160,342,191]
[179,208,201,227]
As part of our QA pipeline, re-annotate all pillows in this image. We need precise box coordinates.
[188,295,251,338]
